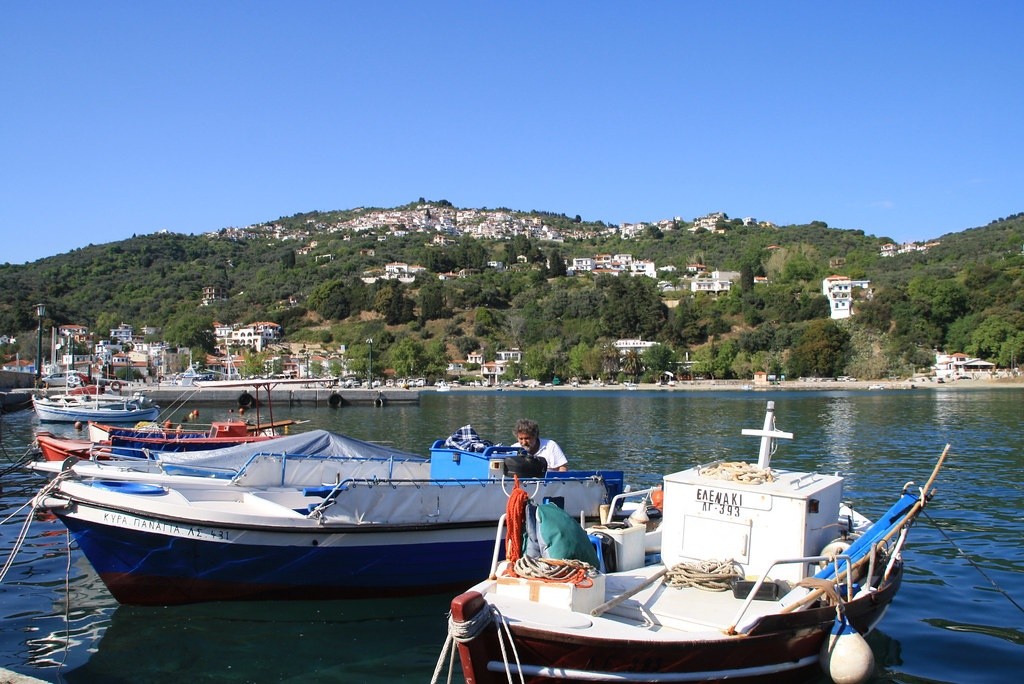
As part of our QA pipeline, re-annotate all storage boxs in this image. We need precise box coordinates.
[585,523,645,573]
[429,440,525,483]
[495,574,606,617]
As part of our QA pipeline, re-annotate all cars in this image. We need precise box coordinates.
[339,376,489,387]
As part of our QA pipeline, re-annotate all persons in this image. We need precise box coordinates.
[508,417,568,472]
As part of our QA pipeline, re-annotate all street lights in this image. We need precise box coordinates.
[365,338,373,389]
[303,351,310,389]
[88,341,94,377]
[69,330,76,370]
[35,301,47,388]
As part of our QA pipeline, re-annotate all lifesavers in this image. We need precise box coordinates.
[110,381,121,391]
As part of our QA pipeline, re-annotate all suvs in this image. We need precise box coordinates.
[40,370,81,389]
[75,373,89,387]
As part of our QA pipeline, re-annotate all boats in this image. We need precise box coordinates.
[33,428,429,461]
[448,400,952,684]
[21,439,527,483]
[30,374,160,423]
[34,473,660,602]
